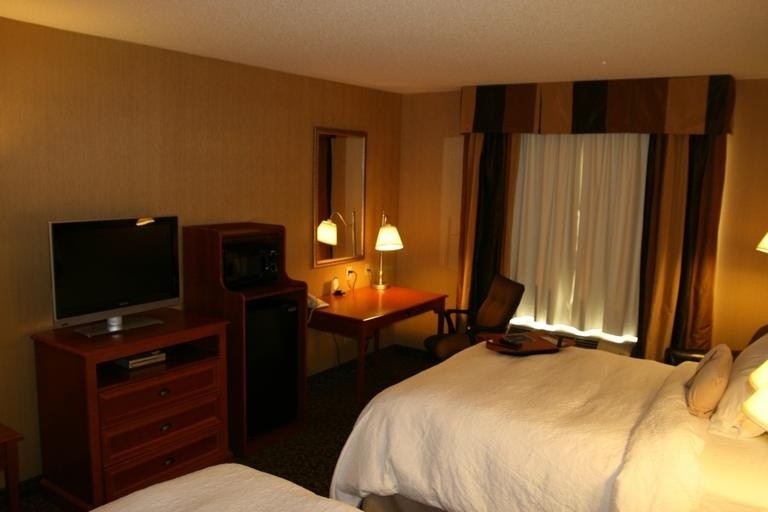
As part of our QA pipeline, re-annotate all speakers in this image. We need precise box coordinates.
[247,297,298,441]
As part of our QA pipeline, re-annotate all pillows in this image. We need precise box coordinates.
[707,326,767,441]
[675,339,736,420]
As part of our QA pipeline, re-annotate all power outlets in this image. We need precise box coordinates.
[344,265,354,280]
[364,263,371,277]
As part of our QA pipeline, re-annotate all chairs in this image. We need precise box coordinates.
[423,271,527,363]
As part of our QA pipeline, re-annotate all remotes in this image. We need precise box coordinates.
[499,335,522,350]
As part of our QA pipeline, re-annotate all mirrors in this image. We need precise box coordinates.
[308,122,370,269]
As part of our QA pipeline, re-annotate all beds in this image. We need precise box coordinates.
[326,323,768,512]
[89,461,380,511]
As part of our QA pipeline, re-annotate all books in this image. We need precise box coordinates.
[484,332,575,356]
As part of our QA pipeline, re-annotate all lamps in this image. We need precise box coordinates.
[369,210,406,291]
[316,208,356,258]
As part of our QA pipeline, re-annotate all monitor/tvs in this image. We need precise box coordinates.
[48,214,183,338]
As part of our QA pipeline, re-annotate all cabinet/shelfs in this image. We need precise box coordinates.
[27,305,234,509]
[177,215,312,449]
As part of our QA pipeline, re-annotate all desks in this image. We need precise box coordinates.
[0,424,24,511]
[296,281,454,409]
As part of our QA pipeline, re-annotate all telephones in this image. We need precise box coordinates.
[307,293,330,309]
